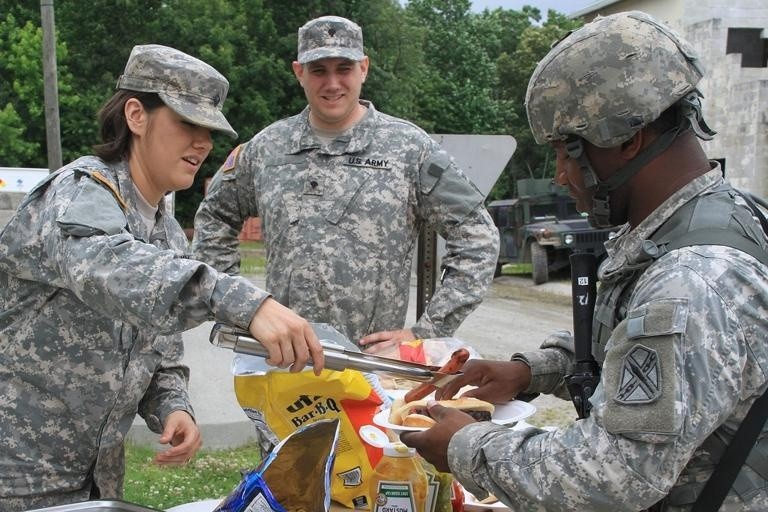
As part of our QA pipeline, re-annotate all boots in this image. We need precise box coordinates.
[487,191,621,283]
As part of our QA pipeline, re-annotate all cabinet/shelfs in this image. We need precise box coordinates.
[358,424,430,512]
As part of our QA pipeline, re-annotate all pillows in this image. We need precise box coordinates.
[523,10,703,149]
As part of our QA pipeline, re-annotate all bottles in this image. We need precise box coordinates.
[297,14,365,63]
[114,43,239,142]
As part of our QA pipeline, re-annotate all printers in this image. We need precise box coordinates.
[208,322,466,390]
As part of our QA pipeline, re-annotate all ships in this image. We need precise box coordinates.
[405,349,469,404]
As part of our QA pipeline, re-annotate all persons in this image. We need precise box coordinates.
[400,11,767,512]
[1,39,328,512]
[191,15,503,355]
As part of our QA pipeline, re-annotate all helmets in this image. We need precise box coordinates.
[372,399,538,434]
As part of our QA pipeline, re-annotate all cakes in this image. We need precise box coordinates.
[359,425,429,512]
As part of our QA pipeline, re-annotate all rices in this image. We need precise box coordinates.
[388,395,495,426]
